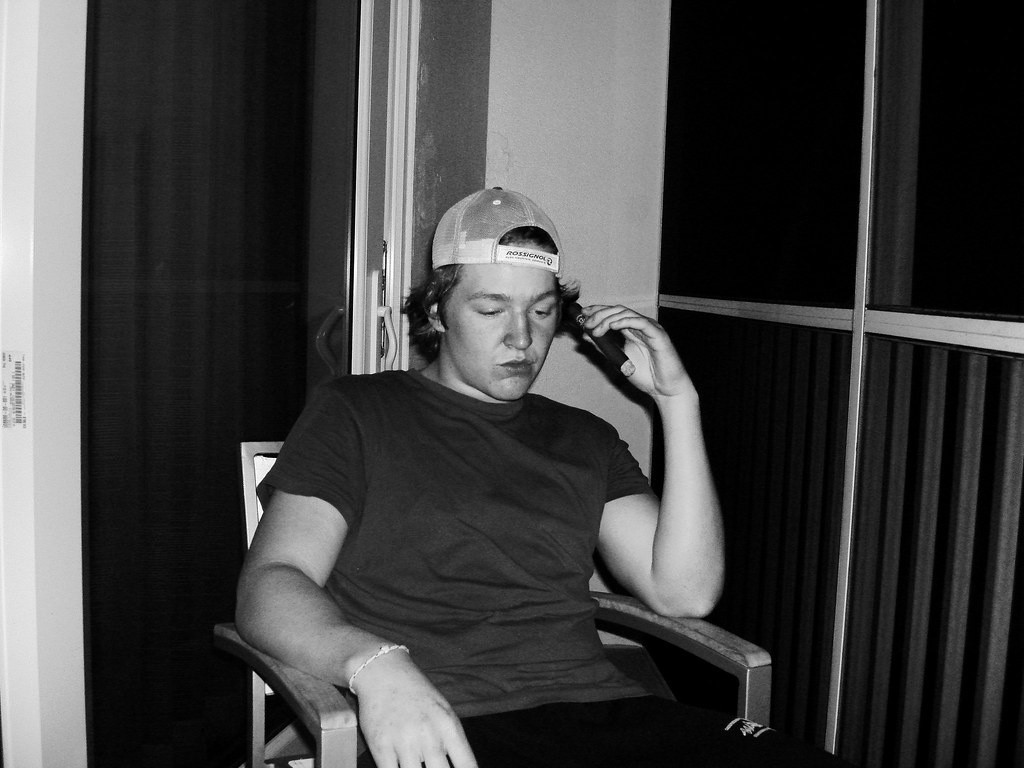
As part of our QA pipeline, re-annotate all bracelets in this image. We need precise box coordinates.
[344,642,417,694]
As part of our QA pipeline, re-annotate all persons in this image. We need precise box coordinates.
[238,186,853,768]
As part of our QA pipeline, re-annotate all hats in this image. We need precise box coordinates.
[432,188,564,279]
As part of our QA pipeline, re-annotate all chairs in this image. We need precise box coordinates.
[212,440,774,768]
